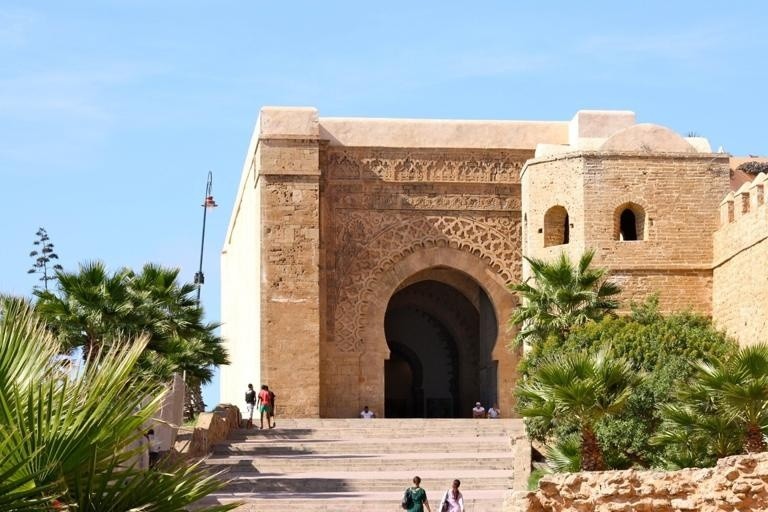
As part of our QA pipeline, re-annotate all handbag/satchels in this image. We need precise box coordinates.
[406,489,413,508]
[441,501,449,512]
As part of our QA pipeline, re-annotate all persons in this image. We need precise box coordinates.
[439,479,466,511]
[403,476,431,512]
[265,385,276,428]
[472,401,486,418]
[486,402,501,418]
[257,385,272,429]
[360,405,376,419]
[245,383,256,429]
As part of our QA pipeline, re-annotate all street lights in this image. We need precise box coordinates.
[195,170,220,313]
